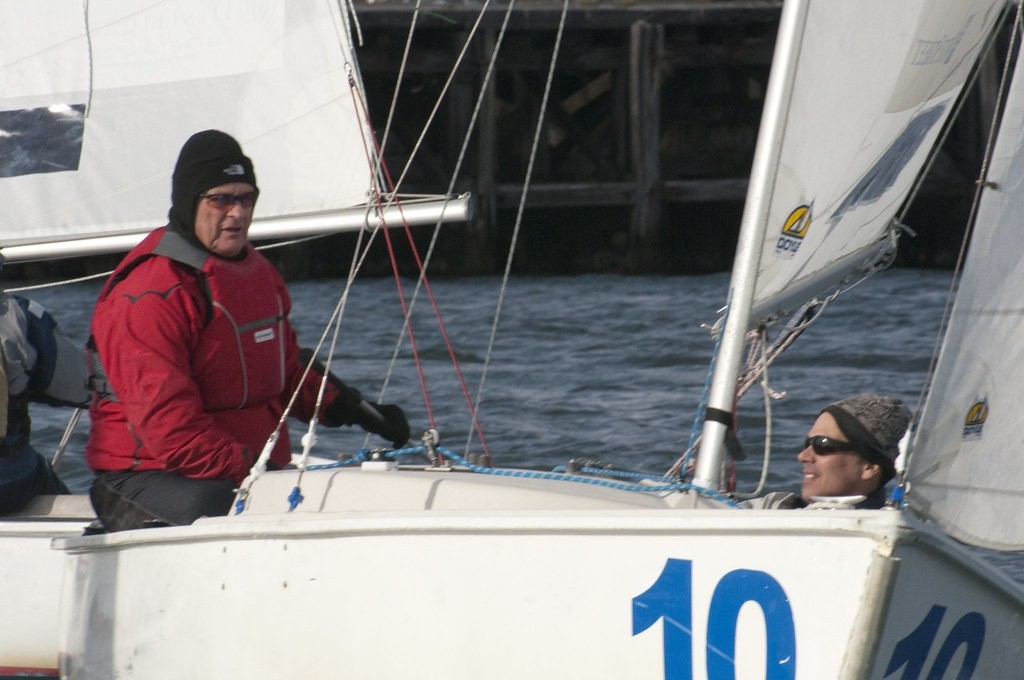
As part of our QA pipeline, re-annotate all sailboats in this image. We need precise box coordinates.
[50,1,1024,680]
[0,1,481,679]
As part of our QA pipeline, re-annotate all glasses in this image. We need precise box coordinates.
[198,191,256,208]
[802,433,862,454]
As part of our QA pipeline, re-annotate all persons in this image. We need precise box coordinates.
[86,128,413,533]
[736,391,914,511]
[0,288,98,517]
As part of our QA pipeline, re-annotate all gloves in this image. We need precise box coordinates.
[356,400,410,450]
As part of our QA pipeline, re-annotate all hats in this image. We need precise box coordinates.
[171,129,259,234]
[819,392,913,475]
